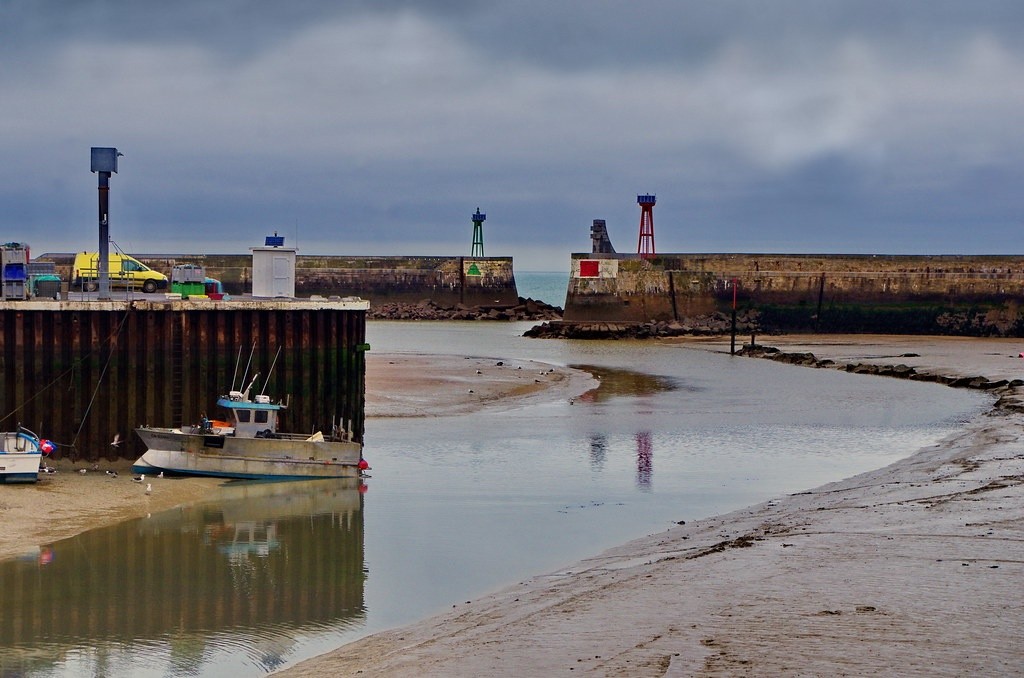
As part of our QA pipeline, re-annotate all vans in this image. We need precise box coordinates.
[72,250,169,293]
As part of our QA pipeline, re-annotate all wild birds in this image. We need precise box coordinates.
[39,434,164,495]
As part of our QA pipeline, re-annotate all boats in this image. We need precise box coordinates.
[0,421,45,484]
[131,338,363,480]
